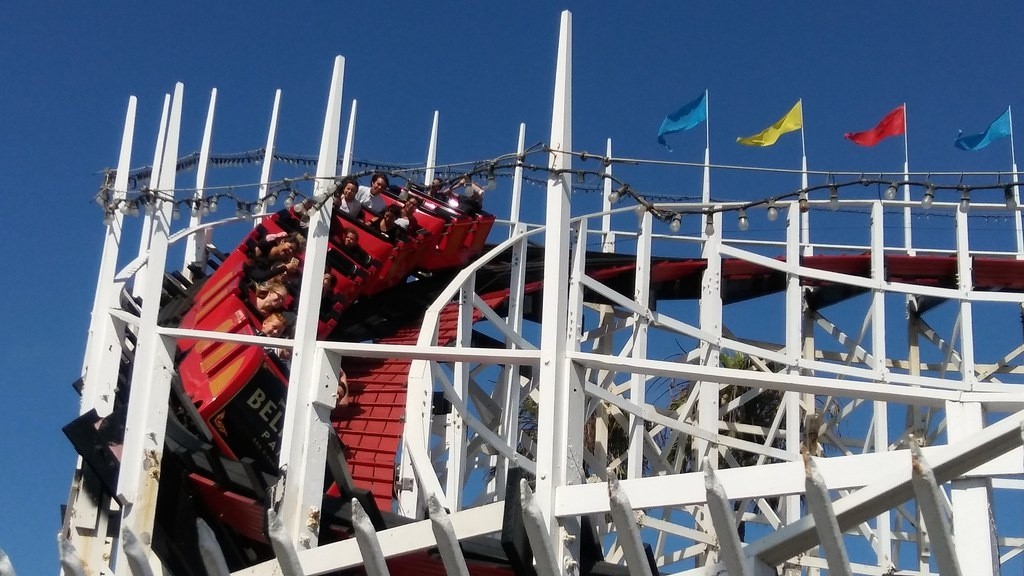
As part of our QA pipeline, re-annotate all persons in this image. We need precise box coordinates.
[354,174,390,213]
[246,176,484,410]
[338,180,360,224]
[377,196,420,233]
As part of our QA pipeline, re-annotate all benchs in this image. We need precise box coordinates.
[175,186,496,393]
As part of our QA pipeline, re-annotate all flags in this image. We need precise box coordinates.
[657,92,706,152]
[736,100,802,146]
[843,106,906,147]
[953,106,1013,152]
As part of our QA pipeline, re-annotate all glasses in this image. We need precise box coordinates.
[409,200,419,208]
[273,291,284,301]
